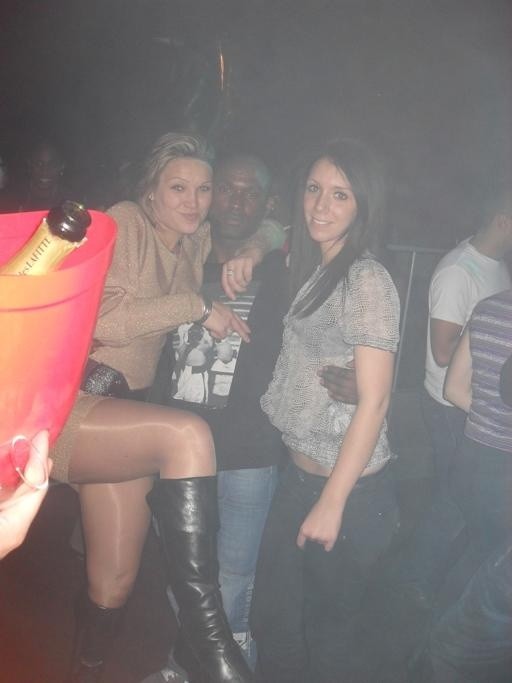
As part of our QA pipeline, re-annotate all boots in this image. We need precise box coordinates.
[153,476,254,682]
[71,590,125,682]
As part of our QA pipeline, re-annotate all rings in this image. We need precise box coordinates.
[227,270,234,275]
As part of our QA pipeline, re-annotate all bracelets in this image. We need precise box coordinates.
[193,293,212,325]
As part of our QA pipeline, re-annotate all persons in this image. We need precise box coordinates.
[145,145,358,682]
[417,184,511,682]
[1,138,80,214]
[260,142,399,682]
[49,132,288,682]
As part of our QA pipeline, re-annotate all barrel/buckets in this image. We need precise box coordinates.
[1,208,117,511]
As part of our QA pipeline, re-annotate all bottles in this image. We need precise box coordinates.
[0,199,91,276]
